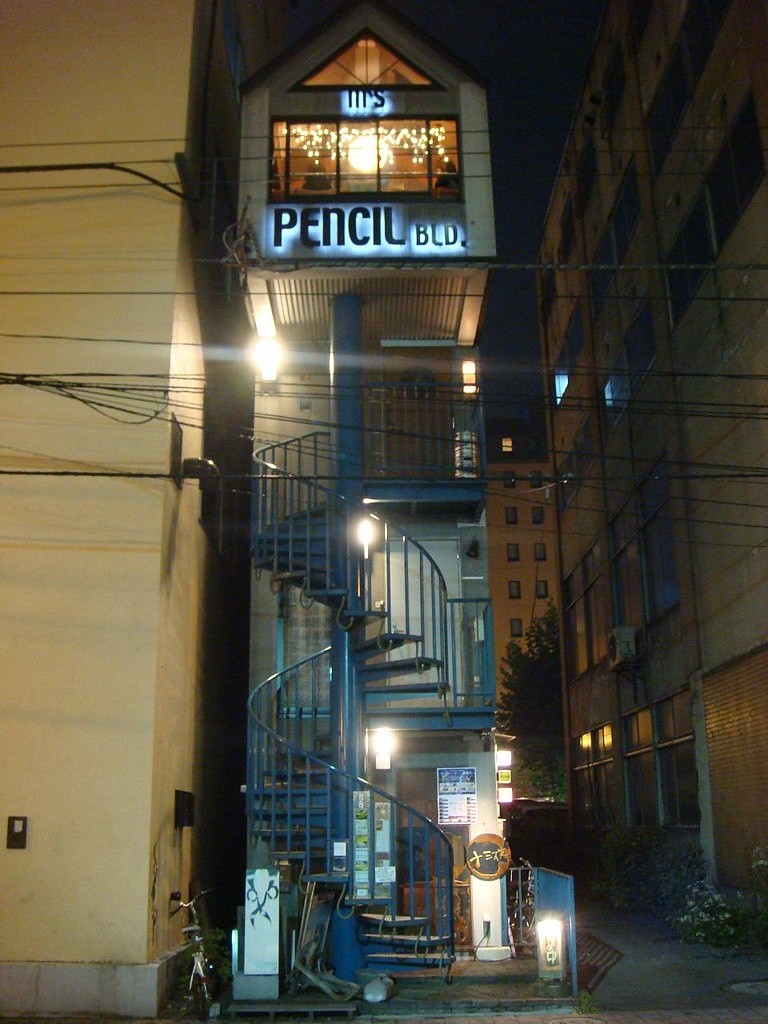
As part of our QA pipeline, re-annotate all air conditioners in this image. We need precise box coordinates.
[607,627,637,672]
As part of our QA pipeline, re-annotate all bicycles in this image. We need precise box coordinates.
[170,887,217,1022]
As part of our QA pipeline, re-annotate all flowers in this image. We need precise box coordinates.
[672,878,756,951]
